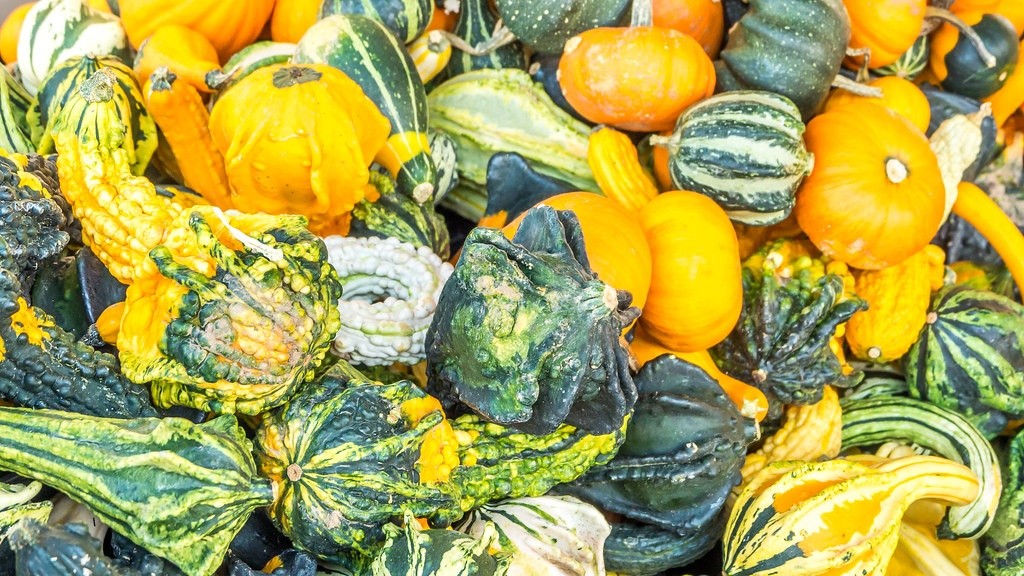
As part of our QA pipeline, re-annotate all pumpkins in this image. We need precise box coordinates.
[0,0,1024,576]
